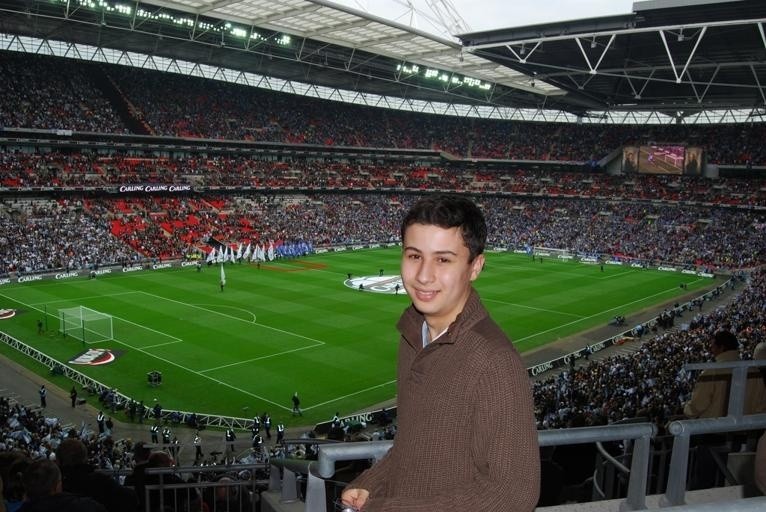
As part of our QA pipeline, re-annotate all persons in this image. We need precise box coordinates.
[359,283,365,290]
[395,284,400,295]
[220,281,224,291]
[337,195,543,510]
[48,363,64,377]
[70,385,77,408]
[1,390,398,511]
[36,319,44,335]
[0,47,766,278]
[38,385,47,407]
[530,277,766,506]
